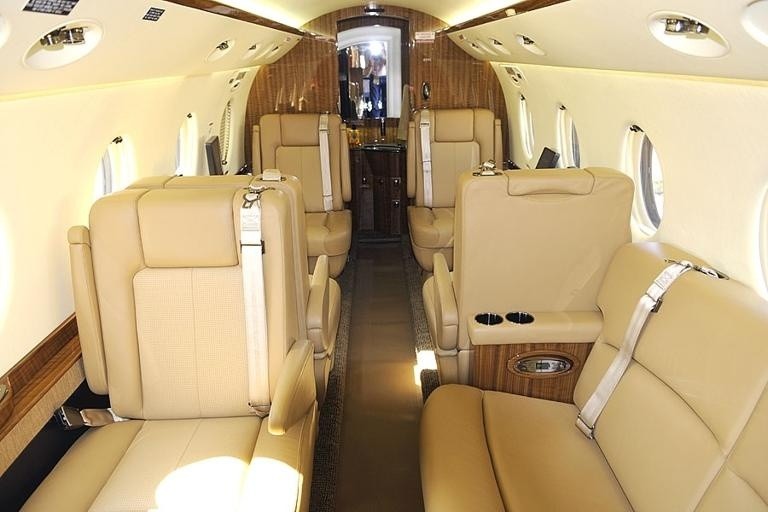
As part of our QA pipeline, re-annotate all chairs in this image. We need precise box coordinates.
[406,108,768,511]
[18,113,351,512]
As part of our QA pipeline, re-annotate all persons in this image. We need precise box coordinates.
[361,43,388,117]
